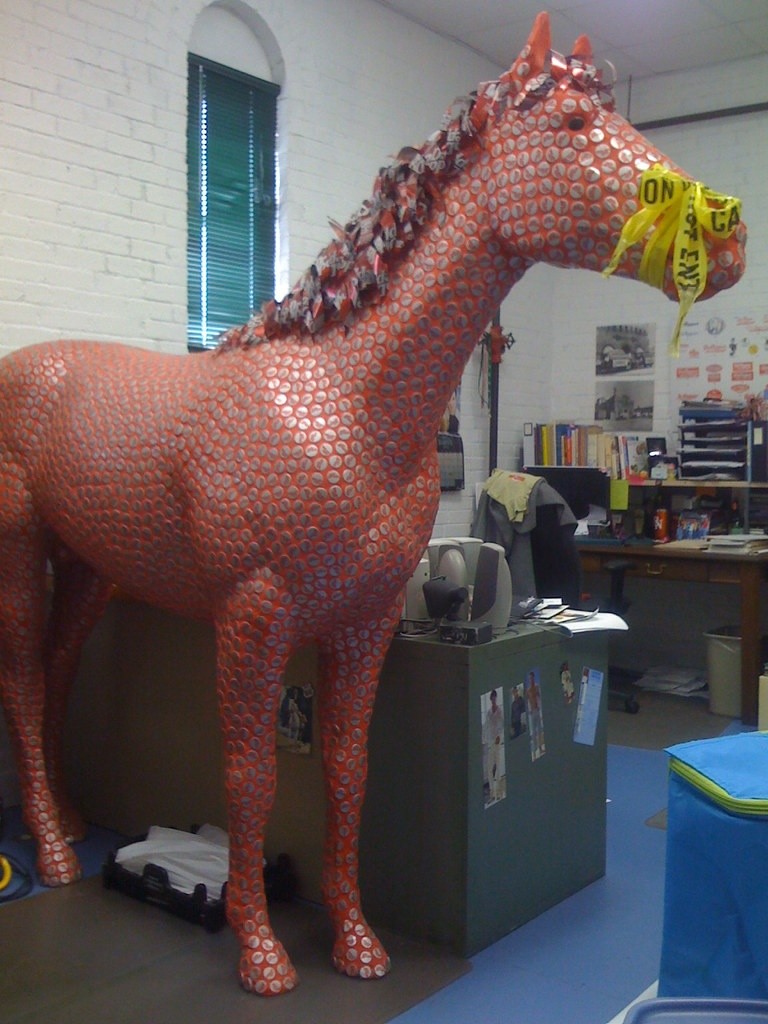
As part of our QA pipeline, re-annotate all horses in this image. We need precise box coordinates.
[0,7,749,997]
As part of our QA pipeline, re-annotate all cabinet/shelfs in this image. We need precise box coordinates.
[69,594,614,961]
[523,407,768,534]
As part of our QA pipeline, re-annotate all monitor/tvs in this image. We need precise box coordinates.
[521,464,611,536]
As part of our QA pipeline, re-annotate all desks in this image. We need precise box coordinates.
[574,538,768,725]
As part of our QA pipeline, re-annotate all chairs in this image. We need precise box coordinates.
[479,473,639,713]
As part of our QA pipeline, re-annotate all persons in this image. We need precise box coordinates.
[479,662,574,803]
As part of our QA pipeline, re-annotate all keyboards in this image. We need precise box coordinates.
[574,537,625,545]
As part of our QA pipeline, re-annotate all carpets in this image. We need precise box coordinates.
[0,867,474,1024]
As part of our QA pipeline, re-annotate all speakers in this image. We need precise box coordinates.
[427,536,513,635]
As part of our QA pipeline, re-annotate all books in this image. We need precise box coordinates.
[524,420,667,482]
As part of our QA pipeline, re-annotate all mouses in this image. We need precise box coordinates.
[628,533,644,541]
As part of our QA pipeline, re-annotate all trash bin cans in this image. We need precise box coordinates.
[702,623,768,718]
